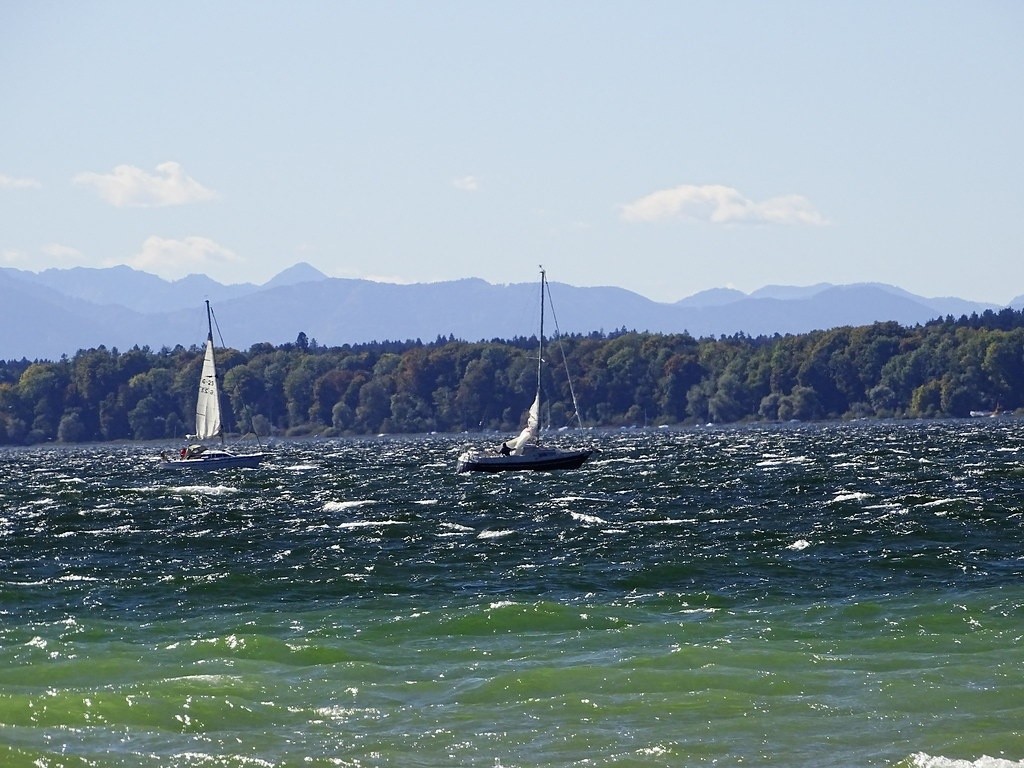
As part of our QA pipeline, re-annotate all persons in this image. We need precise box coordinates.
[491,442,517,457]
[179,443,188,460]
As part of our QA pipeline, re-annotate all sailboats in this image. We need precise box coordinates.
[456,264,601,475]
[159,298,270,469]
